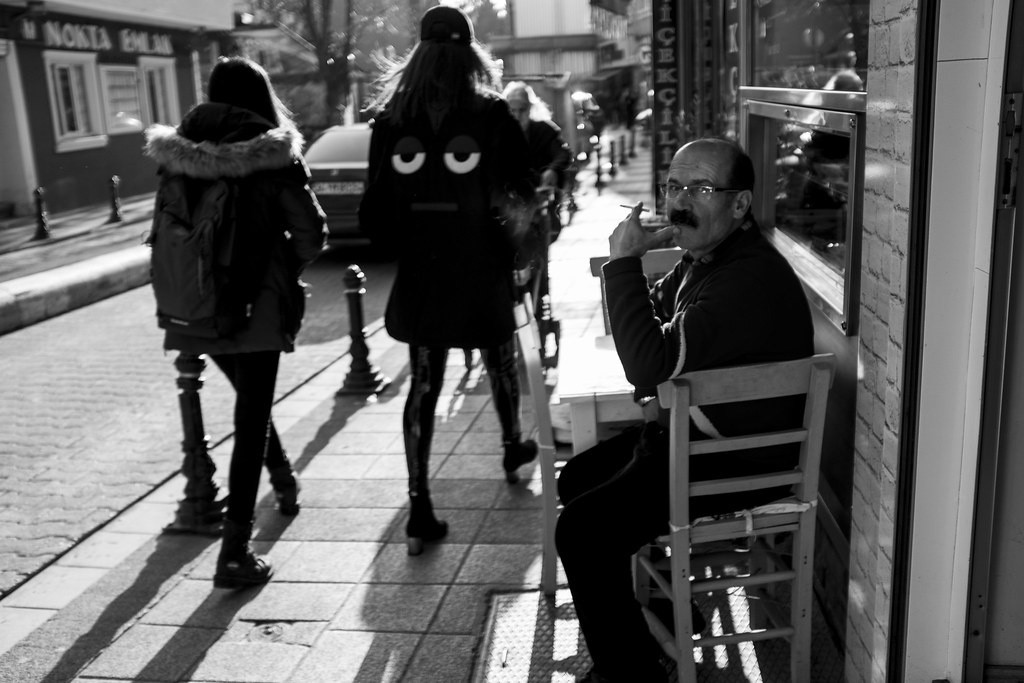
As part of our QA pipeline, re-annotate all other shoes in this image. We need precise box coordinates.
[274,469,301,516]
[575,653,678,683]
[647,599,708,637]
[214,553,274,591]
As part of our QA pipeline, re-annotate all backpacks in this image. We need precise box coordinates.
[149,176,235,336]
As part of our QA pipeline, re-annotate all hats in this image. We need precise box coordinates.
[420,7,471,46]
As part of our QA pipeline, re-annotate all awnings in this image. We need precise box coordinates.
[587,58,641,81]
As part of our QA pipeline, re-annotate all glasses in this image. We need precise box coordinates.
[659,183,739,202]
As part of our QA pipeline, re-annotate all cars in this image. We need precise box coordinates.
[301,118,376,254]
[570,90,607,162]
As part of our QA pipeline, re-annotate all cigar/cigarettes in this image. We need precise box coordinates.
[620,205,650,212]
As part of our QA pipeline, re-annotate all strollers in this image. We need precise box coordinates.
[463,183,579,367]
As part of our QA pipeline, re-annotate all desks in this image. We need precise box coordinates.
[556,335,659,455]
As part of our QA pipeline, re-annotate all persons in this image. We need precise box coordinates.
[623,87,636,129]
[357,6,571,556]
[145,57,328,590]
[554,138,814,683]
[784,72,862,161]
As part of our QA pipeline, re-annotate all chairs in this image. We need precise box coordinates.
[630,354,836,683]
[590,248,686,335]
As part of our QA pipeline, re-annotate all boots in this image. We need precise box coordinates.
[501,432,539,486]
[405,488,448,557]
